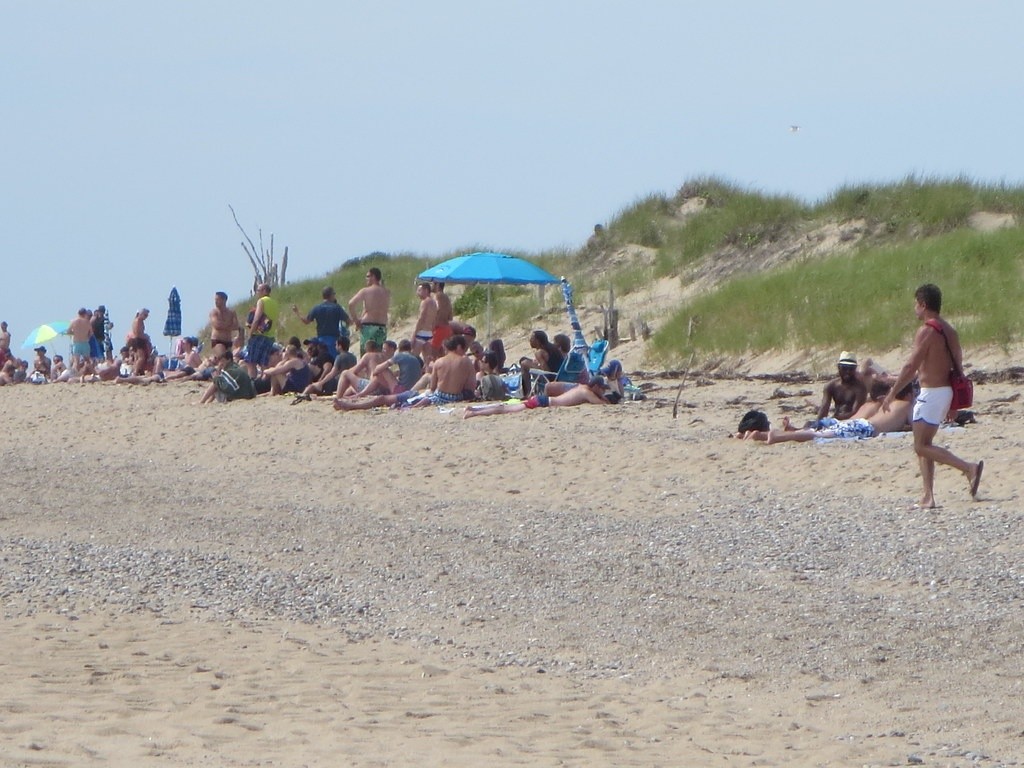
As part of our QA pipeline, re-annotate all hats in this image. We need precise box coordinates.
[588,376,611,389]
[34,346,47,354]
[303,337,319,345]
[600,359,622,377]
[461,327,476,337]
[837,351,860,367]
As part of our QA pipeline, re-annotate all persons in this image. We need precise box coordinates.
[735,350,919,444]
[882,283,984,510]
[0,267,624,419]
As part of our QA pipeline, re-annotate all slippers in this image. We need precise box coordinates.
[909,501,936,512]
[968,461,984,497]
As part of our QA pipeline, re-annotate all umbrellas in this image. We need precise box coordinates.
[418,249,562,340]
[163,285,182,369]
[560,275,591,371]
[23,320,71,355]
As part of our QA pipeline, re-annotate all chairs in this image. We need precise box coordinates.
[528,339,610,399]
[169,342,205,370]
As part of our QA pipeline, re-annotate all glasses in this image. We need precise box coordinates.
[22,365,27,368]
[473,351,478,355]
[366,275,370,278]
[54,360,59,362]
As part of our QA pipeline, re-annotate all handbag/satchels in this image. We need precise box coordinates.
[738,411,770,432]
[951,372,974,409]
